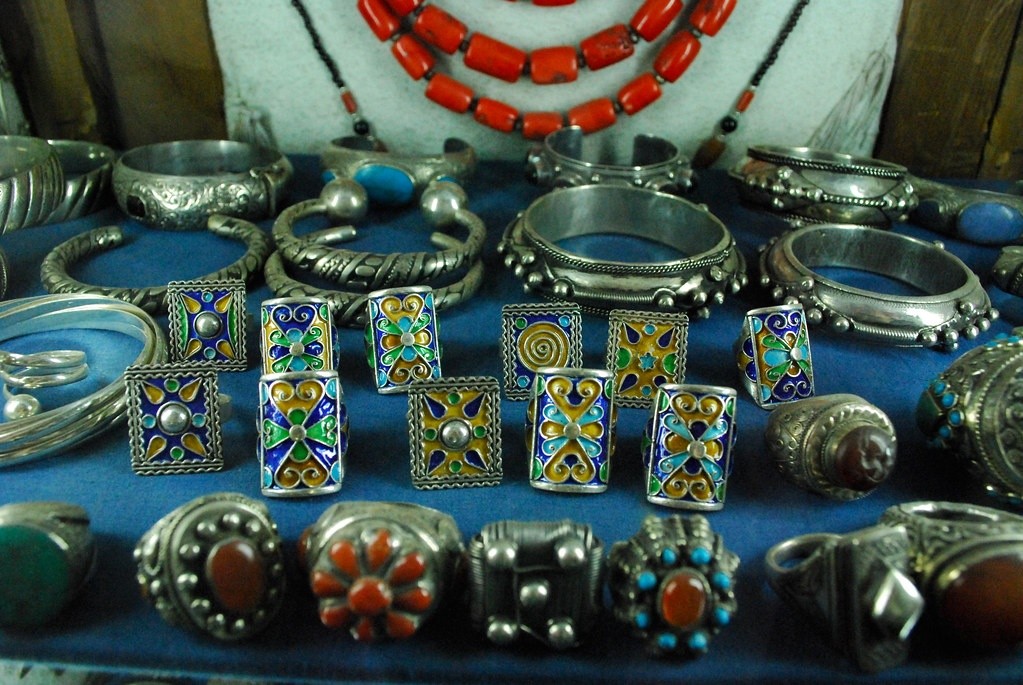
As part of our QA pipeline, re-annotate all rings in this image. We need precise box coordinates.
[498,300,585,406]
[601,508,750,664]
[124,363,224,477]
[766,524,926,680]
[459,517,607,650]
[639,380,741,517]
[361,286,443,395]
[281,495,468,648]
[878,498,1023,672]
[130,492,288,643]
[770,390,898,509]
[257,293,341,377]
[522,365,616,495]
[606,306,688,408]
[404,374,502,491]
[2,497,106,639]
[252,366,350,496]
[911,331,1022,518]
[159,275,249,369]
[732,308,818,410]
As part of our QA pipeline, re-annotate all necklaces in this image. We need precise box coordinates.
[284,2,808,174]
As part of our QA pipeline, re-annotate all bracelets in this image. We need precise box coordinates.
[0,295,167,470]
[0,132,1022,351]
